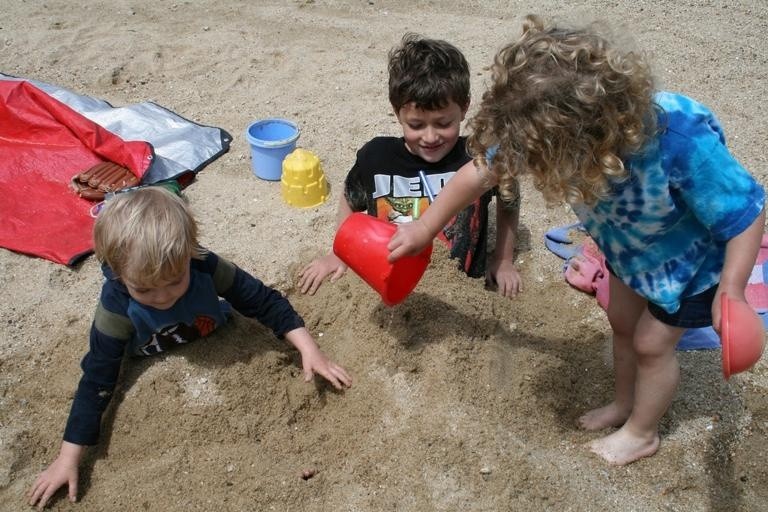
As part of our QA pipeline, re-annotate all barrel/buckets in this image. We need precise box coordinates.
[333,213,433,306]
[245,118,300,181]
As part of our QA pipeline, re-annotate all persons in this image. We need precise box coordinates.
[385,13,767,465]
[25,184,352,508]
[297,30,525,299]
